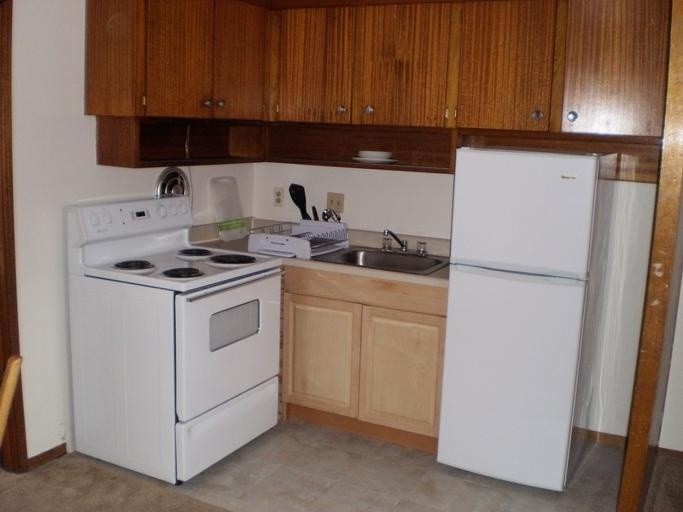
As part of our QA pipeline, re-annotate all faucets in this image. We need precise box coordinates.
[384,228,408,252]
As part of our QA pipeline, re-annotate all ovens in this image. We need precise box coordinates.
[173,268,281,425]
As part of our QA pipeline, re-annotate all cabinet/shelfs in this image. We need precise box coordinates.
[281,267,448,455]
[264,1,453,173]
[83,1,267,169]
[451,1,671,182]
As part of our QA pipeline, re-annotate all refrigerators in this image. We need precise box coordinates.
[435,143,619,494]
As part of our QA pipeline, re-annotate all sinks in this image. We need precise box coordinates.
[340,248,450,276]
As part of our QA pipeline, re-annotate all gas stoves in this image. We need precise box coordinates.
[80,245,283,293]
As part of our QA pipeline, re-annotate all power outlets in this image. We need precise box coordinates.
[326,192,346,215]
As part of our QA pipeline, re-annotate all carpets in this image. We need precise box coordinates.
[1,450,231,512]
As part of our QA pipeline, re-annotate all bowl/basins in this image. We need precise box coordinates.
[357,150,393,159]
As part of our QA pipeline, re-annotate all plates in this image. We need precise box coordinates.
[351,157,399,164]
